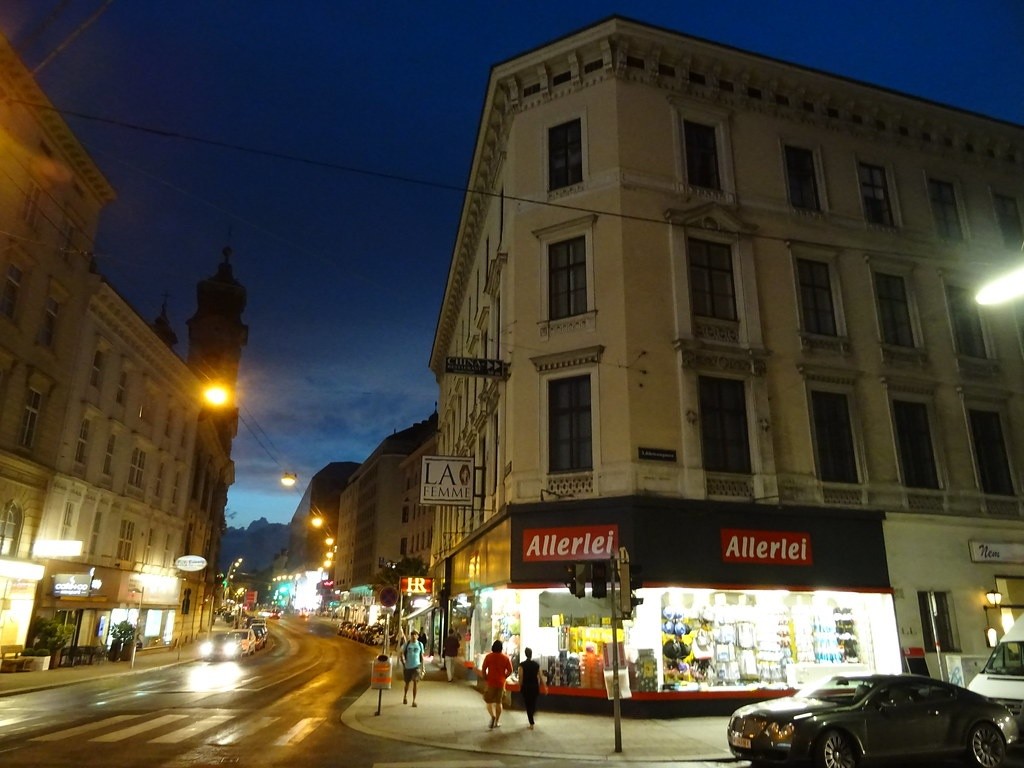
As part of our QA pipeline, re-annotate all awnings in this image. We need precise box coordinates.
[400,603,434,621]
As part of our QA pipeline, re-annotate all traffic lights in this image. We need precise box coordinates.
[621,564,643,613]
[591,563,607,598]
[566,563,586,598]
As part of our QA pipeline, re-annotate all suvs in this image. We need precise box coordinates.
[225,629,256,656]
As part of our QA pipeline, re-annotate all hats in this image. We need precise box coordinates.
[664,605,690,675]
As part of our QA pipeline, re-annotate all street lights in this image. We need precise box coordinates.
[128,586,143,669]
[226,558,243,587]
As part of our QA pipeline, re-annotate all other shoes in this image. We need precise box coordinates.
[488,716,496,729]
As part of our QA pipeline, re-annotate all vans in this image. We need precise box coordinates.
[967,615,1024,741]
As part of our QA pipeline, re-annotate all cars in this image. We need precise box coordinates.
[338,621,384,645]
[215,606,269,650]
[201,633,243,662]
[727,672,1018,768]
[257,608,284,619]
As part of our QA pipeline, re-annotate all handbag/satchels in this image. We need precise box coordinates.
[689,604,719,681]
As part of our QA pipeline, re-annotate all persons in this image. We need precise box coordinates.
[445,628,460,682]
[417,627,427,662]
[517,647,548,729]
[482,640,513,728]
[399,631,425,707]
